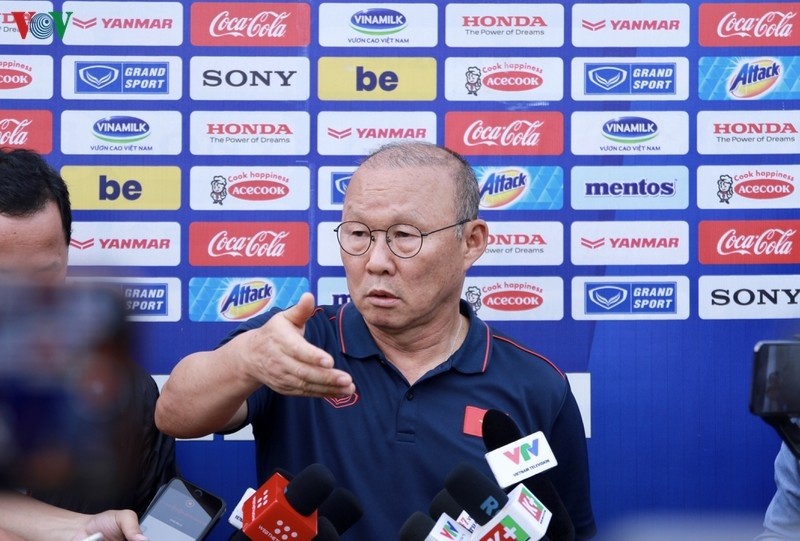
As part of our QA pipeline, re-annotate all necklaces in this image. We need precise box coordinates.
[447,317,463,359]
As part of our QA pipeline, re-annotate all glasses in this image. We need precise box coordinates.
[334,218,471,259]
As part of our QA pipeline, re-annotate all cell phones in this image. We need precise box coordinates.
[137,479,225,540]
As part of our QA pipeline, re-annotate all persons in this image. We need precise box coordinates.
[155,140,597,541]
[753,417,800,541]
[0,145,176,541]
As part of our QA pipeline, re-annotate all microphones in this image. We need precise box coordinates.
[220,409,575,541]
